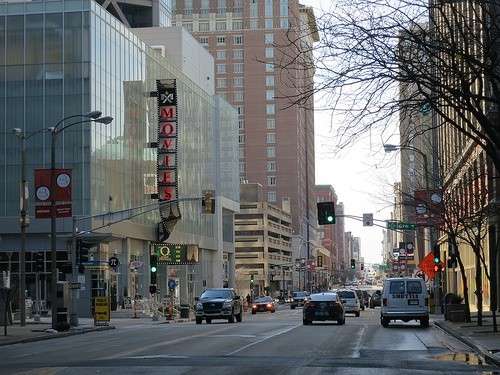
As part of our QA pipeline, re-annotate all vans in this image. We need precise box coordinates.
[376,278,431,328]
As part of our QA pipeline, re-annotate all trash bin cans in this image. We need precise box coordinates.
[444,293,457,321]
[57,307,67,323]
[180,304,190,319]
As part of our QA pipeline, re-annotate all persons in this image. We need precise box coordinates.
[246,294,250,306]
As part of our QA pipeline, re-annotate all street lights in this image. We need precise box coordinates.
[51,110,114,329]
[11,127,57,326]
[310,247,325,293]
[384,220,408,277]
[298,240,316,291]
[384,144,441,314]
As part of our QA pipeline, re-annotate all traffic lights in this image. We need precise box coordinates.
[80,238,89,262]
[361,264,364,270]
[250,275,254,286]
[434,265,441,271]
[34,252,42,271]
[351,259,355,269]
[433,245,440,263]
[149,286,156,294]
[317,202,335,225]
[151,255,157,284]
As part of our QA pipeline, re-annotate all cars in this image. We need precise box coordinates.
[331,288,381,317]
[193,287,243,325]
[303,293,346,325]
[289,291,309,309]
[341,273,383,287]
[250,296,276,314]
